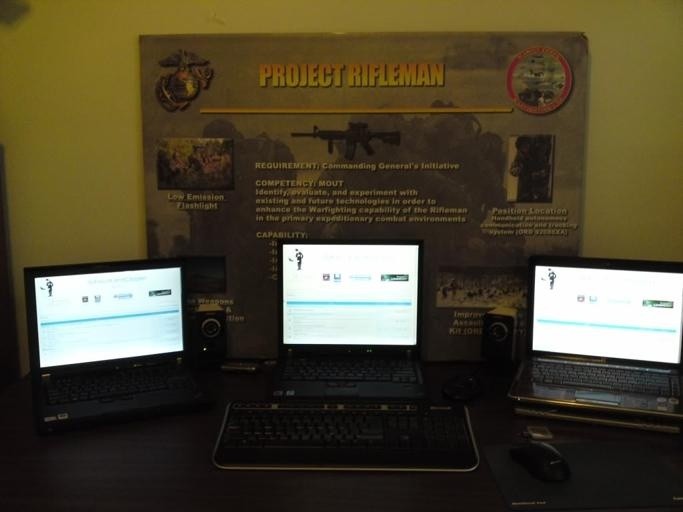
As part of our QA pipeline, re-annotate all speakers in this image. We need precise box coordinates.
[480,313,514,369]
[181,255,226,364]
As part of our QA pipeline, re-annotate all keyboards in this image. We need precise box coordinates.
[210,392,480,474]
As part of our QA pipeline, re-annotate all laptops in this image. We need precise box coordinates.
[23,256,210,427]
[505,253,682,416]
[270,237,427,398]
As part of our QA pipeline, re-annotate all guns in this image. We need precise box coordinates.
[291,121,402,161]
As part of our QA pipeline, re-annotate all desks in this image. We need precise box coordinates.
[0,353,682,512]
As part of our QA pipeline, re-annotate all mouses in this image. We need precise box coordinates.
[511,439,568,479]
